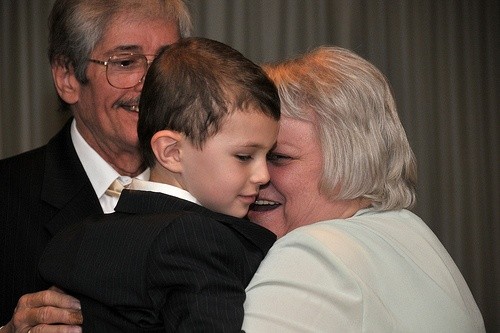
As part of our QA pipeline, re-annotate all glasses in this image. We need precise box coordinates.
[89,53,157,89]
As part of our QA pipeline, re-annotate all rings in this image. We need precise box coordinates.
[26,329,31,332]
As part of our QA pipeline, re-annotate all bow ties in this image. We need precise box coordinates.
[105,180,124,198]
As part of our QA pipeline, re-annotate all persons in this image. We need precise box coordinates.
[241,44,485,333]
[0,0,193,333]
[36,36,277,333]
